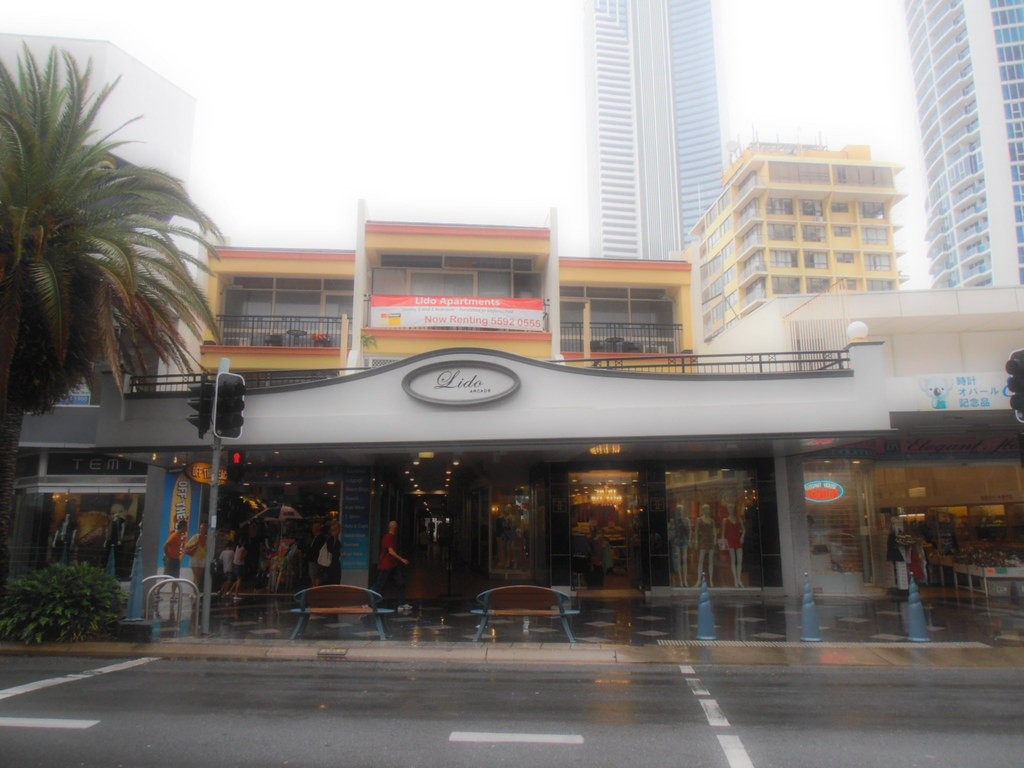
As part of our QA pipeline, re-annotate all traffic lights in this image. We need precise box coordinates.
[212,372,245,440]
[228,448,244,469]
[1006,350,1024,411]
[185,372,215,438]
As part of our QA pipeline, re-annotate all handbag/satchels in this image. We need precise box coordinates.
[184,545,198,556]
[239,551,247,561]
[317,543,333,568]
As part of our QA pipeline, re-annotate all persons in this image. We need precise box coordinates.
[361,520,413,612]
[285,540,306,594]
[668,503,746,588]
[52,513,77,565]
[308,519,349,586]
[428,517,452,562]
[498,504,521,569]
[154,520,248,600]
[100,513,125,580]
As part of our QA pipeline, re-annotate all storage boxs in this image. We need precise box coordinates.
[1012,580,1024,597]
[979,578,1012,597]
[609,537,625,546]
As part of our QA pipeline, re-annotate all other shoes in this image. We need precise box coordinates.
[152,590,160,599]
[169,596,180,602]
[233,597,242,600]
[308,614,328,621]
[189,592,205,598]
[226,592,233,599]
[217,590,227,600]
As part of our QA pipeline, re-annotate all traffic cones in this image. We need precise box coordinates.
[696,571,717,640]
[120,548,143,620]
[104,544,116,577]
[799,572,821,642]
[907,571,931,643]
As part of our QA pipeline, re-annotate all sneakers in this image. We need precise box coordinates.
[358,604,370,620]
[397,604,413,610]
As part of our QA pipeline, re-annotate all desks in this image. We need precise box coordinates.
[952,562,1024,597]
[287,330,307,347]
[605,338,624,352]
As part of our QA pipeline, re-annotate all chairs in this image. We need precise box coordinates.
[265,335,283,346]
[623,342,639,352]
[590,340,607,352]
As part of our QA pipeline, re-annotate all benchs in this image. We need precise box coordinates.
[470,585,581,643]
[290,584,396,640]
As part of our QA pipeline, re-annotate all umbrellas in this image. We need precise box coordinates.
[252,504,303,539]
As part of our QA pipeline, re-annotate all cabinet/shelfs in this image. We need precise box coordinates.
[572,533,629,577]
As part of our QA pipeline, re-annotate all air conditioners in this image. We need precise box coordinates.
[643,345,667,354]
[223,338,251,346]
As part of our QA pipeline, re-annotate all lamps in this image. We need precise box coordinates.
[846,321,870,342]
